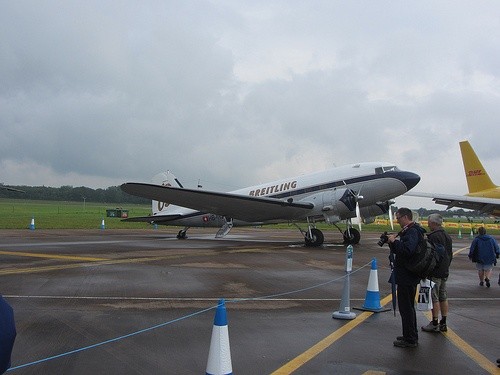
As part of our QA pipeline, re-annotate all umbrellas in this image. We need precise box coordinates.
[388,247,396,317]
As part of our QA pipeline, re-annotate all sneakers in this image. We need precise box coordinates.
[420,320,442,334]
[439,322,448,333]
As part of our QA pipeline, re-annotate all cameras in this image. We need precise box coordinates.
[377,231,390,247]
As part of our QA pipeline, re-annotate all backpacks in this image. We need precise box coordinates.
[412,225,440,280]
[433,242,452,279]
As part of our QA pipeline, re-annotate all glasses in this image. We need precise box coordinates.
[395,215,406,220]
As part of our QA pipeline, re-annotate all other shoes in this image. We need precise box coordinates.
[484,278,491,288]
[480,281,484,287]
[396,335,405,341]
[393,339,420,349]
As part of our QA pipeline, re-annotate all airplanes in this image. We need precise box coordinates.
[118,164,421,249]
[402,139,500,223]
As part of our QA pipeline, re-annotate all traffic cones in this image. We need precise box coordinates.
[352,259,391,313]
[100,218,107,230]
[204,297,234,375]
[29,217,36,230]
[457,228,463,239]
[468,230,473,239]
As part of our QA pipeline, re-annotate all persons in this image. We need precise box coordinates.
[469,225,500,287]
[385,207,428,348]
[423,213,453,333]
